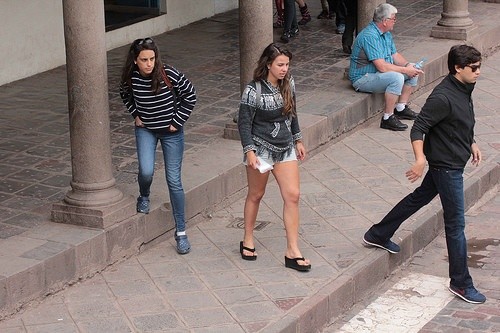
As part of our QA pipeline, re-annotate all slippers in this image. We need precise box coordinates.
[136,195,150,214]
[174,231,190,253]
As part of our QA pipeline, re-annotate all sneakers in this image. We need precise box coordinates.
[381,114,408,130]
[394,104,420,119]
[363,233,400,253]
[336,24,346,33]
[449,280,485,303]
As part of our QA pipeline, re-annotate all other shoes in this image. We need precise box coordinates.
[316,11,329,19]
[281,29,291,41]
[343,44,352,54]
[290,26,300,36]
[329,13,337,18]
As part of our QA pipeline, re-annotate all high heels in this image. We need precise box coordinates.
[285,256,311,270]
[240,240,257,260]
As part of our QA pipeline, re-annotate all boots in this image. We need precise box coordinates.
[298,3,311,25]
[273,9,285,27]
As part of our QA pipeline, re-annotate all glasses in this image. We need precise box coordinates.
[466,62,483,72]
[385,17,397,22]
[134,38,153,47]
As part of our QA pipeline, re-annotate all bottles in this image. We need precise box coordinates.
[405,58,428,81]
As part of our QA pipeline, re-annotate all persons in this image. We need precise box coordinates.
[120,39,197,255]
[349,3,422,132]
[363,44,488,305]
[238,42,313,273]
[271,0,358,55]
